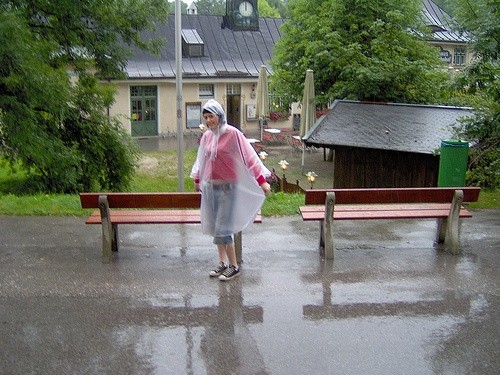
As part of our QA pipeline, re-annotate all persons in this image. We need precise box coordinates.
[190,99,271,281]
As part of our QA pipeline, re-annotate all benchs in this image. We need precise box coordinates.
[299,186,480,261]
[80,188,262,252]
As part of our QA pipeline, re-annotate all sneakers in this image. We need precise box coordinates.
[208,261,228,277]
[219,263,240,280]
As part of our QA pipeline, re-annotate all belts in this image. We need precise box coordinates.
[213,183,234,191]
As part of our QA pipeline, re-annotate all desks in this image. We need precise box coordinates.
[246,138,259,155]
[291,135,309,152]
[264,128,282,146]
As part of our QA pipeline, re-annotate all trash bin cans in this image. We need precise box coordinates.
[437,139,469,187]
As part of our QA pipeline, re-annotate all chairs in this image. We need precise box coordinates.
[253,128,319,159]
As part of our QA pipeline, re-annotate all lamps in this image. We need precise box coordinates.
[279,160,290,169]
[306,171,318,183]
[259,150,269,161]
